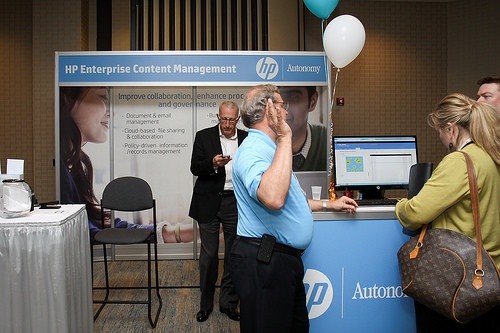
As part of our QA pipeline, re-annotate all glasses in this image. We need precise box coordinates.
[273,100,289,111]
[218,116,240,123]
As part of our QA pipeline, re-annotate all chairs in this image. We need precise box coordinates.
[92,176,162,329]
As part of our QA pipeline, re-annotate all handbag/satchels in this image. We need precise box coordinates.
[397,152,500,324]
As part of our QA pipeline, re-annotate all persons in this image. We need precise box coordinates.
[60,87,223,245]
[395,94,500,333]
[188,101,248,322]
[228,86,358,333]
[277,85,327,172]
[476,76,500,115]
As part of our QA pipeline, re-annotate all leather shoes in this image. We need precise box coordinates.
[219,306,240,321]
[197,308,213,322]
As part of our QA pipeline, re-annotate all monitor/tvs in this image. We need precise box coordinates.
[332,135,418,200]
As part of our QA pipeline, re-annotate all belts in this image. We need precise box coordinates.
[240,236,302,257]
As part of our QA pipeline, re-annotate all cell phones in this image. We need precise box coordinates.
[222,155,230,158]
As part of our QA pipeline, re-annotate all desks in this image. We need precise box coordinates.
[0,203,94,333]
[300,204,416,333]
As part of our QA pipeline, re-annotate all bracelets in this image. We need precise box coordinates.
[175,223,182,243]
[322,201,326,212]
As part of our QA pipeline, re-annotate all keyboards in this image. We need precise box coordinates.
[356,199,400,205]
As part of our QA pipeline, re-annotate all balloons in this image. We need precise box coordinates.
[303,0,339,21]
[323,14,366,69]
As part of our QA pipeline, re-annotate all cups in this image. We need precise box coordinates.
[311,186,322,200]
